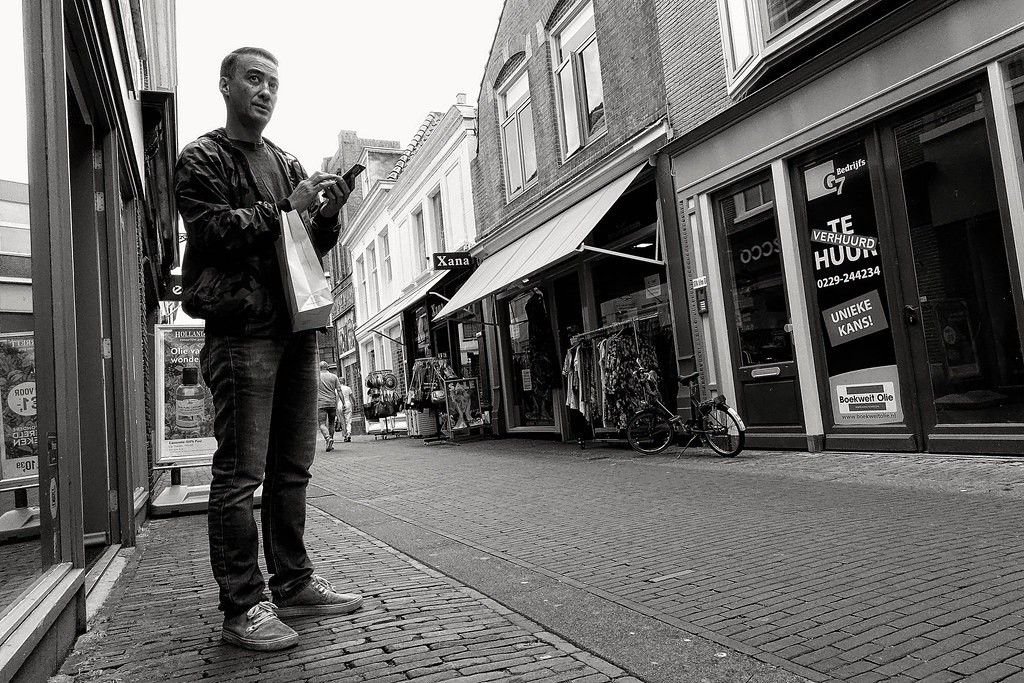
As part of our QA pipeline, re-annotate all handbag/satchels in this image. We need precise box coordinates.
[273,203,332,334]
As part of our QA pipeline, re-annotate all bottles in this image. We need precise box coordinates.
[175,368,204,430]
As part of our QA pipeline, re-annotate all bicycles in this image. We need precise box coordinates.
[626,354,747,460]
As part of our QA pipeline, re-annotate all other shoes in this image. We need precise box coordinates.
[269,575,364,618]
[221,602,299,651]
[325,438,335,451]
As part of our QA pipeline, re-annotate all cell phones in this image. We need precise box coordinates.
[322,164,366,199]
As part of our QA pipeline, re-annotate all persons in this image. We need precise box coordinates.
[334,377,356,441]
[318,361,347,452]
[173,46,357,652]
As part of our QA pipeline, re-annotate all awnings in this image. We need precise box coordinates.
[431,154,665,321]
